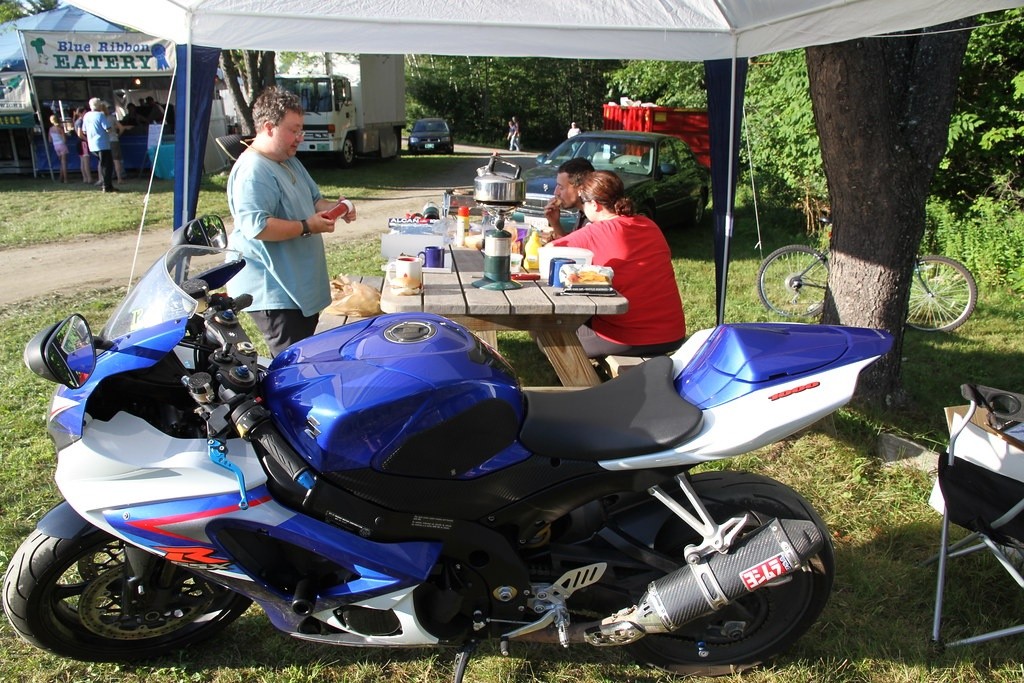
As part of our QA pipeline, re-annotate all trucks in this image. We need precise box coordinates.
[273,47,408,168]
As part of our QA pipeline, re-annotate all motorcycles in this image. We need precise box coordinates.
[0,213,898,683]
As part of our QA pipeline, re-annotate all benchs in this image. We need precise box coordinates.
[600,352,666,378]
[313,274,385,334]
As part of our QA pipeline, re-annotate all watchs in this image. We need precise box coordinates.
[301,220,311,238]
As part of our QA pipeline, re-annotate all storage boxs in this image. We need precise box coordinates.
[928,404,1023,559]
[380,232,445,259]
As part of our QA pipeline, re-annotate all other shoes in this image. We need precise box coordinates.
[84,178,95,183]
[103,188,119,194]
[94,180,103,186]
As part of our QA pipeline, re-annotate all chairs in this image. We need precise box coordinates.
[593,152,613,166]
[930,382,1024,659]
[640,154,651,168]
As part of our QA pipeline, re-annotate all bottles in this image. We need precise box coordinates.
[457,206,468,247]
[525,228,543,269]
[322,199,354,221]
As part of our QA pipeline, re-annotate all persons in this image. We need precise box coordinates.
[567,121,582,152]
[92,99,129,186]
[119,93,168,137]
[544,169,685,382]
[506,116,520,151]
[74,97,120,191]
[49,114,73,184]
[224,85,356,360]
[543,156,595,240]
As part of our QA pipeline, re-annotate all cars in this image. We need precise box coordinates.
[407,118,456,155]
[520,130,711,238]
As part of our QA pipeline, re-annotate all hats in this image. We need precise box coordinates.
[100,101,112,108]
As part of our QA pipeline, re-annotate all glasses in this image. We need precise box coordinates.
[580,198,598,204]
[269,122,306,140]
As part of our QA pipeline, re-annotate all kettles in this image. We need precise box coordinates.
[474,155,525,203]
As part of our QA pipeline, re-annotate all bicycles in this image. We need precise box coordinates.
[756,216,978,335]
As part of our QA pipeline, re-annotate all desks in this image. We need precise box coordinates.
[380,244,629,389]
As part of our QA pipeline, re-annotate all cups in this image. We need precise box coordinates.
[386,257,421,291]
[511,254,523,273]
[549,258,576,287]
[418,245,445,268]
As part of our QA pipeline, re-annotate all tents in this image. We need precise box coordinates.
[0,4,224,178]
